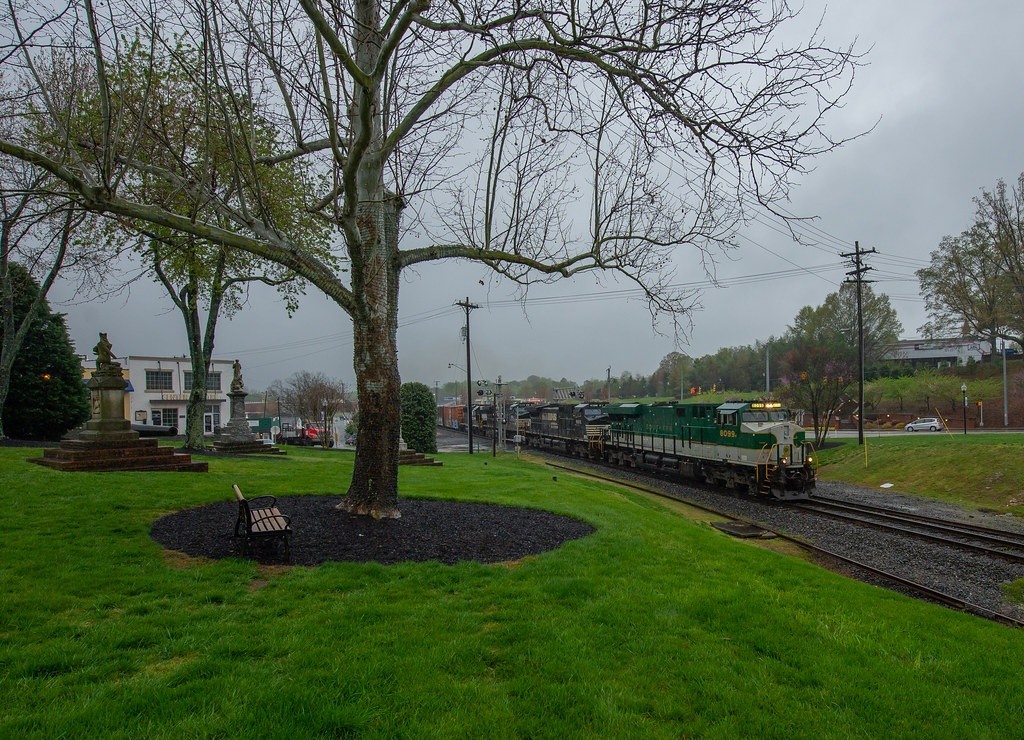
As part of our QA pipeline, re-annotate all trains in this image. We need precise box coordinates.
[434,394,820,504]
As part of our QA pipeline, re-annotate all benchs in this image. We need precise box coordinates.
[232,484,293,557]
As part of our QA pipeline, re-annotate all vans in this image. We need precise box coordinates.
[905,418,942,431]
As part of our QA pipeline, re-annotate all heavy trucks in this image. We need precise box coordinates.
[279,416,335,449]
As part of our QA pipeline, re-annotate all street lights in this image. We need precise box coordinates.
[448,363,467,375]
[960,382,968,436]
